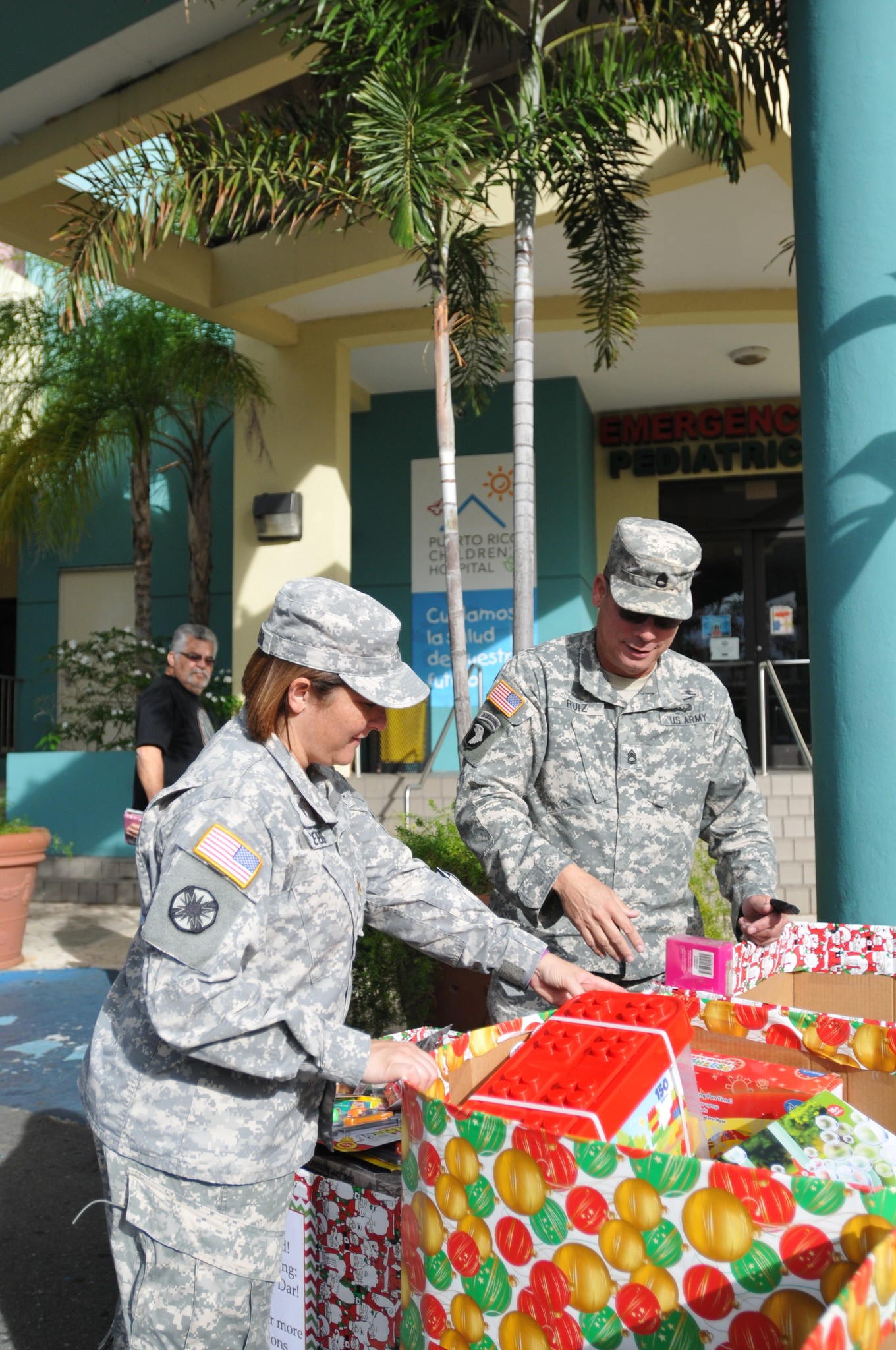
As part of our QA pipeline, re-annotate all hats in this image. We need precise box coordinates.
[258,576,430,708]
[603,517,703,619]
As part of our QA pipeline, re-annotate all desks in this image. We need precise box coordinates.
[264,1145,402,1350]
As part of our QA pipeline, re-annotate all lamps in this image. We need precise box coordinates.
[731,346,770,365]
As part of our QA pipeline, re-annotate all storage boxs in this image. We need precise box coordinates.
[462,991,710,1160]
[666,934,733,995]
[709,636,740,661]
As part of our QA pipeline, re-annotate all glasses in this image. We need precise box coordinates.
[618,605,684,630]
[172,651,217,667]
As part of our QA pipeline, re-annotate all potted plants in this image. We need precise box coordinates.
[0,788,74,971]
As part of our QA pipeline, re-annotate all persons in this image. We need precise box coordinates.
[134,622,227,812]
[452,516,787,1028]
[76,575,629,1350]
